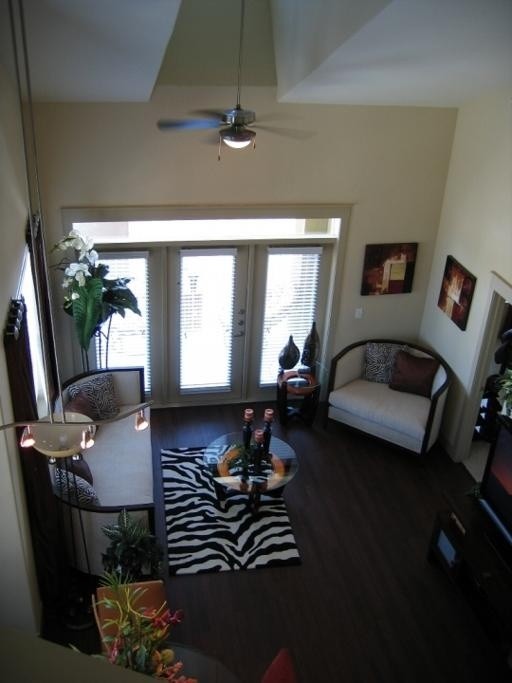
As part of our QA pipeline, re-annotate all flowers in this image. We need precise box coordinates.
[47,226,143,374]
[91,571,197,683]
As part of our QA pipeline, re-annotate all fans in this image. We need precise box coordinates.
[157,0,319,161]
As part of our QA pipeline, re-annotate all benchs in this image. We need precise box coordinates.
[50,367,157,584]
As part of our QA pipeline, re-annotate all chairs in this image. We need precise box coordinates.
[325,339,453,466]
[95,580,174,652]
[262,648,296,682]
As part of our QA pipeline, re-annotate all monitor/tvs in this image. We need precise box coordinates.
[479,423,512,538]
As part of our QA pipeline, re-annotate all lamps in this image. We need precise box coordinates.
[80,427,95,448]
[28,295,95,457]
[137,411,150,428]
[218,1,256,161]
[20,425,35,447]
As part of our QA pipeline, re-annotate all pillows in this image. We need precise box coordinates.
[65,391,100,433]
[387,350,440,398]
[69,373,121,421]
[61,454,93,485]
[55,469,101,505]
[363,342,409,383]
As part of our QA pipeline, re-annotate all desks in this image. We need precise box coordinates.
[90,646,244,682]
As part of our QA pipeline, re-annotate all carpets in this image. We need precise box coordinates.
[160,447,301,576]
